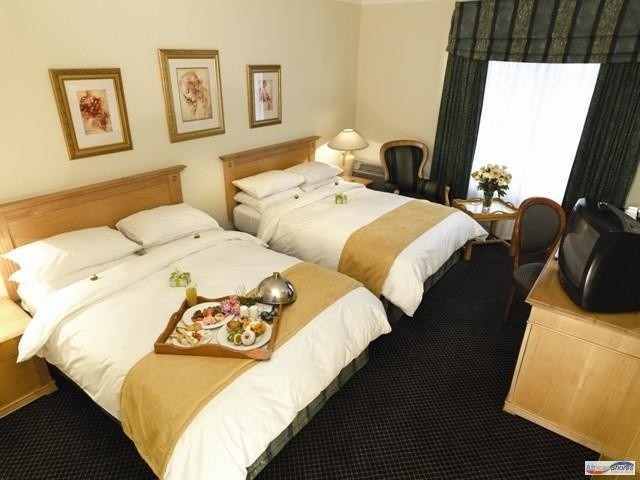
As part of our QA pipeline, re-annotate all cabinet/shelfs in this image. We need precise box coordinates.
[503,236,640,461]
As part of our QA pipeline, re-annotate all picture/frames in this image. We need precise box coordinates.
[156,45,226,147]
[48,62,135,160]
[245,62,285,128]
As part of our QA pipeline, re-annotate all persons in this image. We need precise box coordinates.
[258,80,275,113]
[80,91,112,134]
[179,72,212,119]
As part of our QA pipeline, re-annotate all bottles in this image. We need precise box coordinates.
[240,305,260,320]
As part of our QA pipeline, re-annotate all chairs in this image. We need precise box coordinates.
[504,193,565,332]
[376,136,452,209]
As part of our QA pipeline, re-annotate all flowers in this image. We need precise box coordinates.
[471,164,511,205]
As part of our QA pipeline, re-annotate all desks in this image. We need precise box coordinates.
[454,195,520,260]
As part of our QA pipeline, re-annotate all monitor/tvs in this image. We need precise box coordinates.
[557,197,640,313]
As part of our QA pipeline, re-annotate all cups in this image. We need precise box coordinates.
[185,288,198,306]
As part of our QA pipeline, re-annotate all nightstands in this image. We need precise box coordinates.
[0,287,60,421]
[344,176,375,191]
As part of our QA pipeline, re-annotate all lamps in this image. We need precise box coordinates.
[326,128,371,178]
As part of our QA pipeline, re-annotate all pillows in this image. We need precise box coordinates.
[0,195,217,310]
[232,158,344,216]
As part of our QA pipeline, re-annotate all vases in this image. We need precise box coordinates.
[481,190,493,208]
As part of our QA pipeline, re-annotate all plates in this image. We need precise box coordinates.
[171,328,212,348]
[181,301,234,329]
[216,318,272,350]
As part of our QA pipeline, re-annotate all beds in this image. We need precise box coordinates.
[219,133,491,320]
[0,163,397,478]
